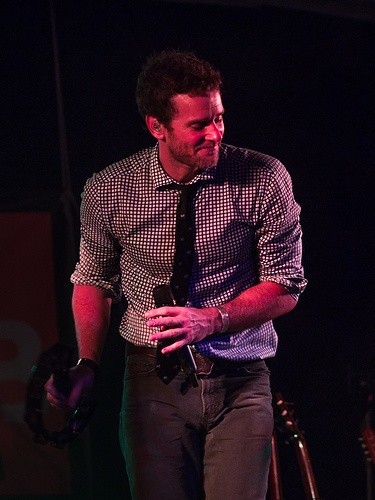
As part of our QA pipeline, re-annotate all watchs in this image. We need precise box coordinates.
[212,306,229,335]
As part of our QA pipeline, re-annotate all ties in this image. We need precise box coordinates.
[154,176,206,376]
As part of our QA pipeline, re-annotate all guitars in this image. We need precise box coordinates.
[274,390,320,500]
[357,426,375,462]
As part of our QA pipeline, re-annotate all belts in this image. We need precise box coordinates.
[123,337,211,360]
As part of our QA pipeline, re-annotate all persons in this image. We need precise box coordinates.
[44,50,310,500]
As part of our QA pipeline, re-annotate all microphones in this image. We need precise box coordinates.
[152,284,200,389]
